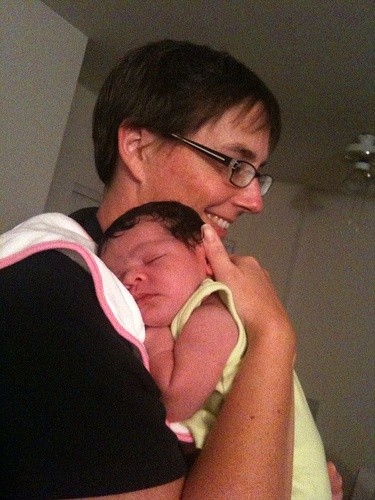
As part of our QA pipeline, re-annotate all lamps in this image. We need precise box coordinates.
[342,134,375,185]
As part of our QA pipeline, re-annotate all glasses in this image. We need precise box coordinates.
[150,128,272,197]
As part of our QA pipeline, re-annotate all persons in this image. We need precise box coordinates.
[0,40,346,499]
[94,202,335,500]
